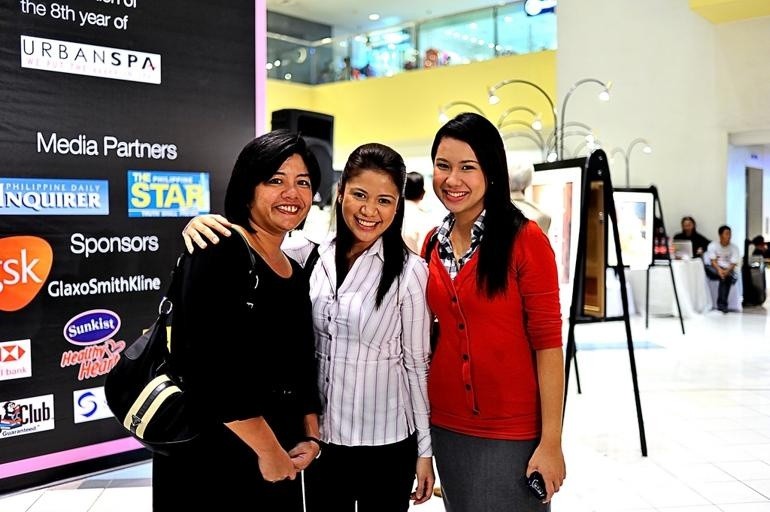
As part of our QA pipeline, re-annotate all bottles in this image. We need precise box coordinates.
[653,236,668,260]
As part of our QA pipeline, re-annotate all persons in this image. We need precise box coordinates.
[420,113,567,512]
[181,144,435,512]
[673,215,708,255]
[702,226,740,313]
[154,128,321,512]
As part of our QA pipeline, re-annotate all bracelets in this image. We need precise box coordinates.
[306,437,321,459]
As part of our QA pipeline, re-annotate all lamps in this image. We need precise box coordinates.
[436,79,652,187]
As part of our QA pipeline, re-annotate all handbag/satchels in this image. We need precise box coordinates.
[105,314,205,458]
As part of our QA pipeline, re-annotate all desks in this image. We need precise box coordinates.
[629,257,713,317]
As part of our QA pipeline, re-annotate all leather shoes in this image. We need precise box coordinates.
[729,270,738,284]
[718,305,729,314]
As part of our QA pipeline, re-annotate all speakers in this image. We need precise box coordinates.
[270,109,333,206]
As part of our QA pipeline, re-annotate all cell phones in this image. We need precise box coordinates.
[527,463,549,502]
